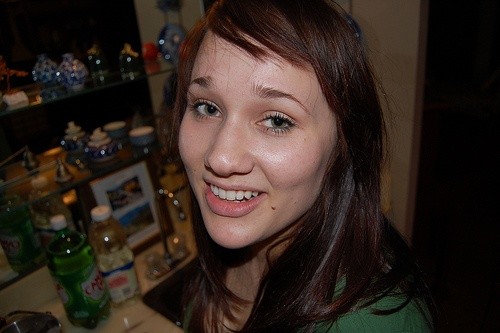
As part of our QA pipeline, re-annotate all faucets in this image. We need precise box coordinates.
[144,189,186,280]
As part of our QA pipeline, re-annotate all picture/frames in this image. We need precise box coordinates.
[74,153,175,258]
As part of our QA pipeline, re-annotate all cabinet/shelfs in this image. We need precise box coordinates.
[0,0,219,333]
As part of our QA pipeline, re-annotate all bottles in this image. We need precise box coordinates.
[46,216,112,328]
[29,175,75,245]
[0,180,46,273]
[88,208,140,308]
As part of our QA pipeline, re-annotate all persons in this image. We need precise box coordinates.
[166,0,436,332]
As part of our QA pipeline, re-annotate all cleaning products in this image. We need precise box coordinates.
[46,215,111,330]
